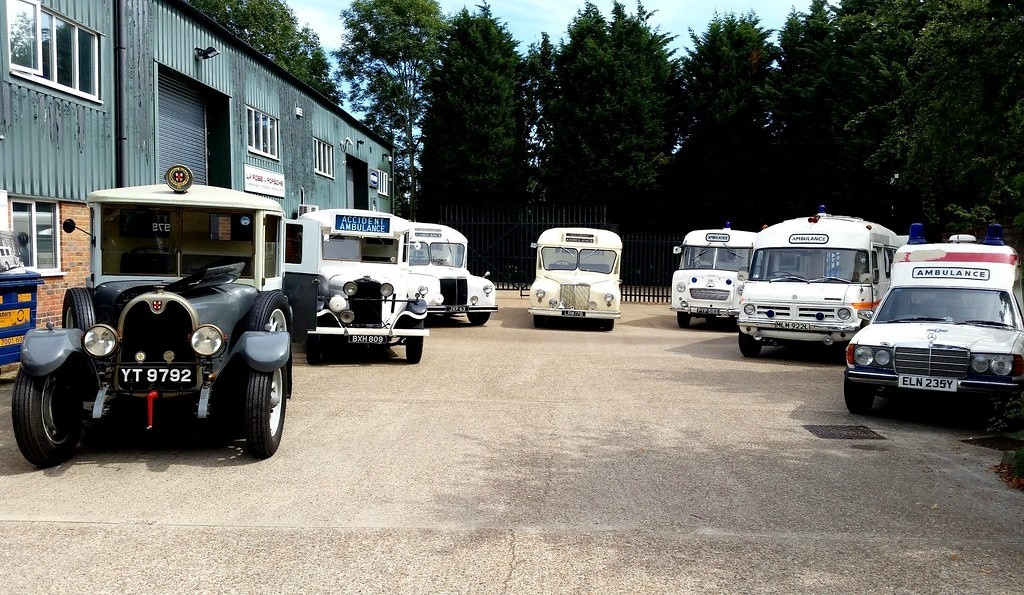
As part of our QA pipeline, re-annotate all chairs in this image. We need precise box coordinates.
[908,303,930,317]
[699,264,717,268]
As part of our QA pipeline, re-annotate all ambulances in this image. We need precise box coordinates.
[411,220,497,328]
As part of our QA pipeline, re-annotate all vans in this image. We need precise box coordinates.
[737,205,899,360]
[665,221,757,331]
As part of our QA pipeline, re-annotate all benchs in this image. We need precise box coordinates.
[121,251,249,277]
[548,263,610,273]
[409,259,448,266]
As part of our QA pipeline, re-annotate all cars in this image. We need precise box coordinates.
[9,163,321,468]
[841,219,1024,415]
[527,226,623,332]
[294,209,430,372]
